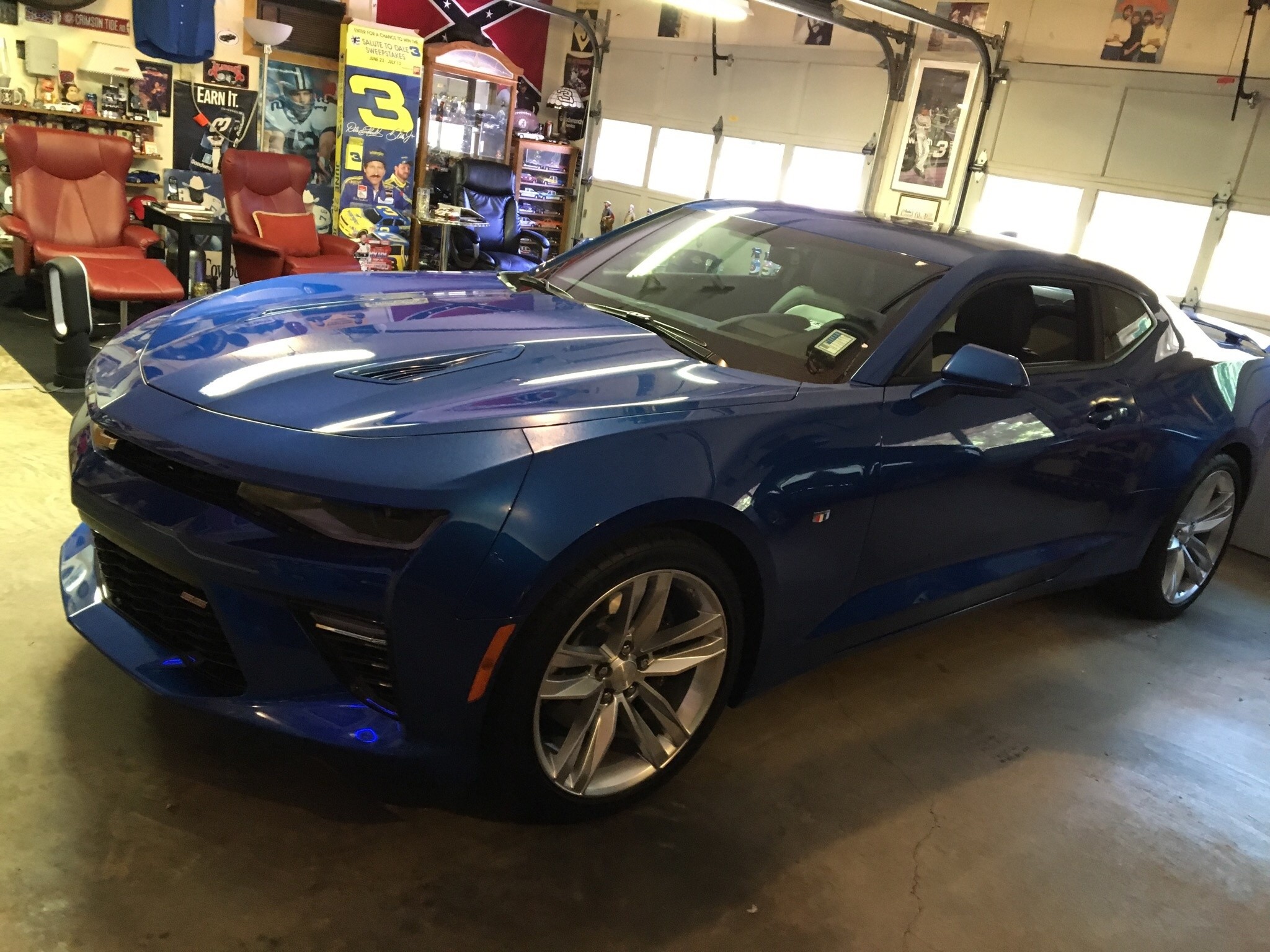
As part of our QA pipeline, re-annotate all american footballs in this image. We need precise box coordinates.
[512,108,538,131]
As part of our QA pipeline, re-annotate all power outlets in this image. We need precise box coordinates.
[180,64,194,80]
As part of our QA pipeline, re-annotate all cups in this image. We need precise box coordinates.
[0,86,22,106]
[544,120,553,142]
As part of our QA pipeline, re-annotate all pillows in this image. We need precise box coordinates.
[252,211,322,258]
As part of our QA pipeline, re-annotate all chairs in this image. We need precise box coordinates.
[448,158,550,273]
[768,247,888,331]
[0,126,184,341]
[221,148,360,284]
[931,283,1039,372]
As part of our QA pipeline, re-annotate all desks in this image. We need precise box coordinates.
[402,209,490,271]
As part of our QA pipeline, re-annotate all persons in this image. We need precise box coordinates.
[383,156,412,209]
[332,146,412,215]
[167,176,223,253]
[600,200,653,236]
[263,63,336,189]
[129,78,167,116]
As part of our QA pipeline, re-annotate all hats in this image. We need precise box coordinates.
[182,175,211,191]
[363,147,388,167]
[394,156,414,167]
[302,189,319,203]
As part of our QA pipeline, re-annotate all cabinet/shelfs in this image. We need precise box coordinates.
[509,137,579,260]
[407,41,524,271]
[142,205,233,309]
[0,105,163,248]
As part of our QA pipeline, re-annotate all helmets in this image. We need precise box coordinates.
[277,65,314,121]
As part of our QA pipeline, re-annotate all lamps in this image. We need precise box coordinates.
[652,0,755,76]
[77,41,144,119]
[546,88,584,143]
[243,18,293,152]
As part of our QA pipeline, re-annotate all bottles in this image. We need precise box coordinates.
[168,176,179,201]
[496,100,506,127]
[118,82,127,119]
[431,93,466,123]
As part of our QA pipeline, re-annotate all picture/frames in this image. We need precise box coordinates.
[890,56,982,200]
[896,193,941,222]
[128,59,173,117]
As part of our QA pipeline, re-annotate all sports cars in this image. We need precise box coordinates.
[58,198,1270,825]
